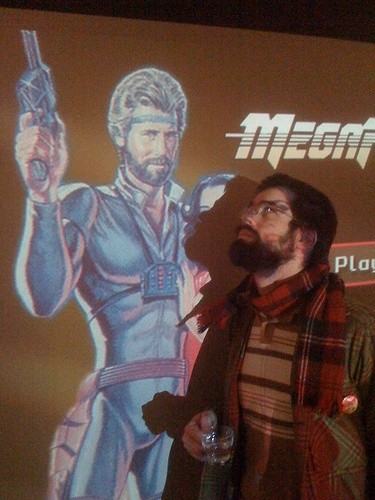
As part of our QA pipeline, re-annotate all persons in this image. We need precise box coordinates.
[181,173,375,500]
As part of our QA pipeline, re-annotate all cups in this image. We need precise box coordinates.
[201,427,234,465]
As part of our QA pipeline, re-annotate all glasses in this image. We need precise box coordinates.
[239,205,309,226]
